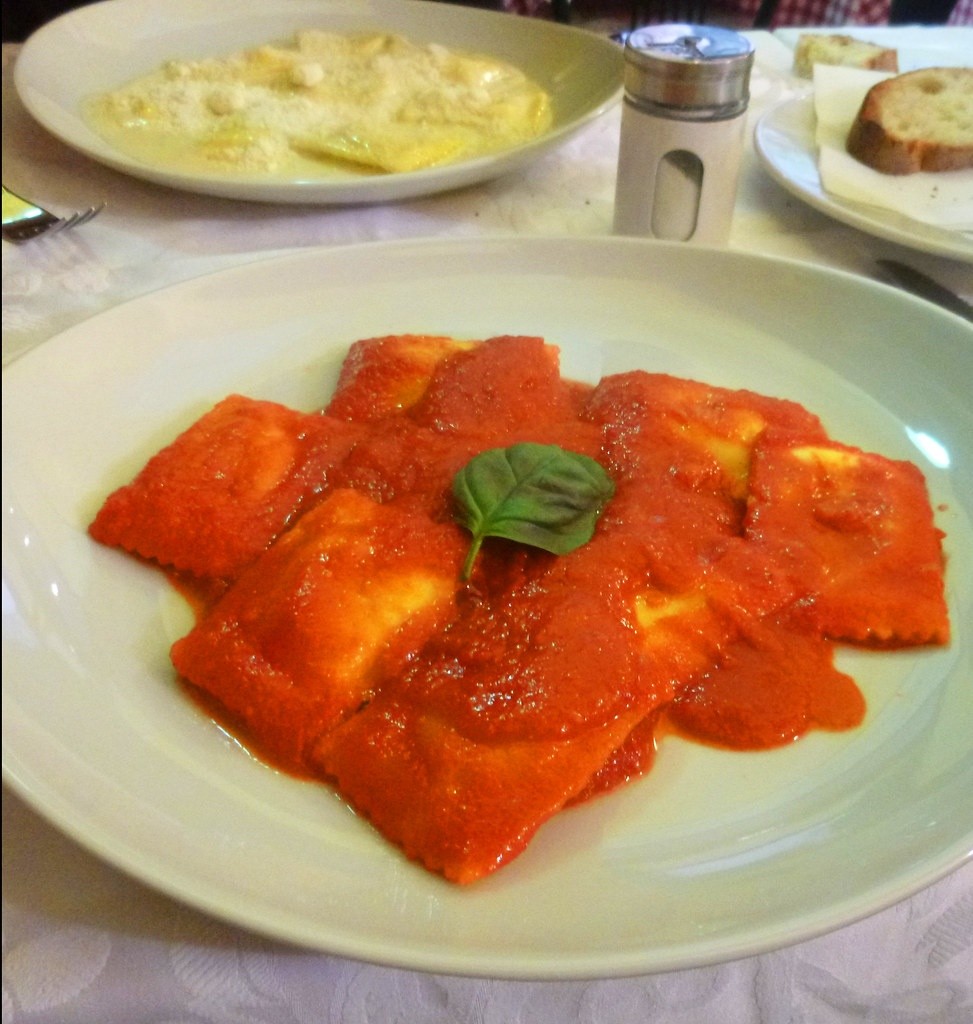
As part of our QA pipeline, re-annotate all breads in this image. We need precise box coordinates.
[846,63,973,177]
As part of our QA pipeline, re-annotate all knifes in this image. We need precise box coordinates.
[875,259,972,324]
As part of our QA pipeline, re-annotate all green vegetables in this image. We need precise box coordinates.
[450,440,615,580]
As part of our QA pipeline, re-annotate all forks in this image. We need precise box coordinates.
[4,187,106,240]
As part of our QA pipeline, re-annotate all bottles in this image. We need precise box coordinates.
[614,21,754,243]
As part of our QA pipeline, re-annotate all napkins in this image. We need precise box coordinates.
[810,59,973,233]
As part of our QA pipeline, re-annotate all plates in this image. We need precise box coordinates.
[11,1,628,203]
[3,232,973,981]
[750,73,973,270]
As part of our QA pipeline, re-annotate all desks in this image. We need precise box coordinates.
[2,26,973,1024]
